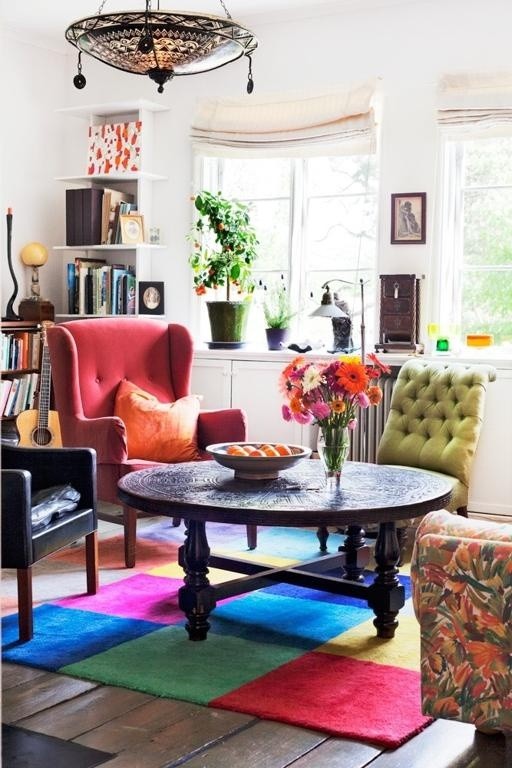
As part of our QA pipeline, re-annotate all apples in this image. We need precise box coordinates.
[228,442,292,458]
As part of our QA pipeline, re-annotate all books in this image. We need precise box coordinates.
[64,186,135,245]
[65,256,137,314]
[0,331,48,422]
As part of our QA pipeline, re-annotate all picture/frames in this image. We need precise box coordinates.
[387,191,429,247]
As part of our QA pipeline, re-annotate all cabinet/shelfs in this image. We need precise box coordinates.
[0,319,55,425]
[49,169,169,320]
[372,271,427,355]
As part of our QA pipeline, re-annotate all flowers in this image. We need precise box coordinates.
[274,350,382,471]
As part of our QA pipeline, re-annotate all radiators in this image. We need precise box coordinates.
[344,373,398,464]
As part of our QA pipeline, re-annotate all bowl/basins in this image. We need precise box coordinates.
[466,334,495,347]
[206,442,314,480]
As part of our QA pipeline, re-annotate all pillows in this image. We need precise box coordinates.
[102,378,210,466]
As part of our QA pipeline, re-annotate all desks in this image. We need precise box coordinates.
[110,455,458,639]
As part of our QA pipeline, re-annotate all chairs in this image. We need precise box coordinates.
[38,316,268,571]
[409,494,512,743]
[371,354,498,567]
[1,435,107,646]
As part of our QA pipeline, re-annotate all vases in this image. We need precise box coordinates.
[310,422,353,477]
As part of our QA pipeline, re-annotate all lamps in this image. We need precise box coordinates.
[315,272,369,373]
[14,240,56,323]
[63,0,260,100]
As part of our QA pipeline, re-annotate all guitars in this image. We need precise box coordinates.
[15,321,62,449]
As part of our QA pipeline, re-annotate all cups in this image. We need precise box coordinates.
[436,335,449,352]
[148,227,160,244]
[427,320,440,336]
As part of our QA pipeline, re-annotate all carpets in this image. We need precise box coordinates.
[1,517,446,750]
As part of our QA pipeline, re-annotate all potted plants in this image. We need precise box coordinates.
[173,181,260,342]
[259,290,303,350]
